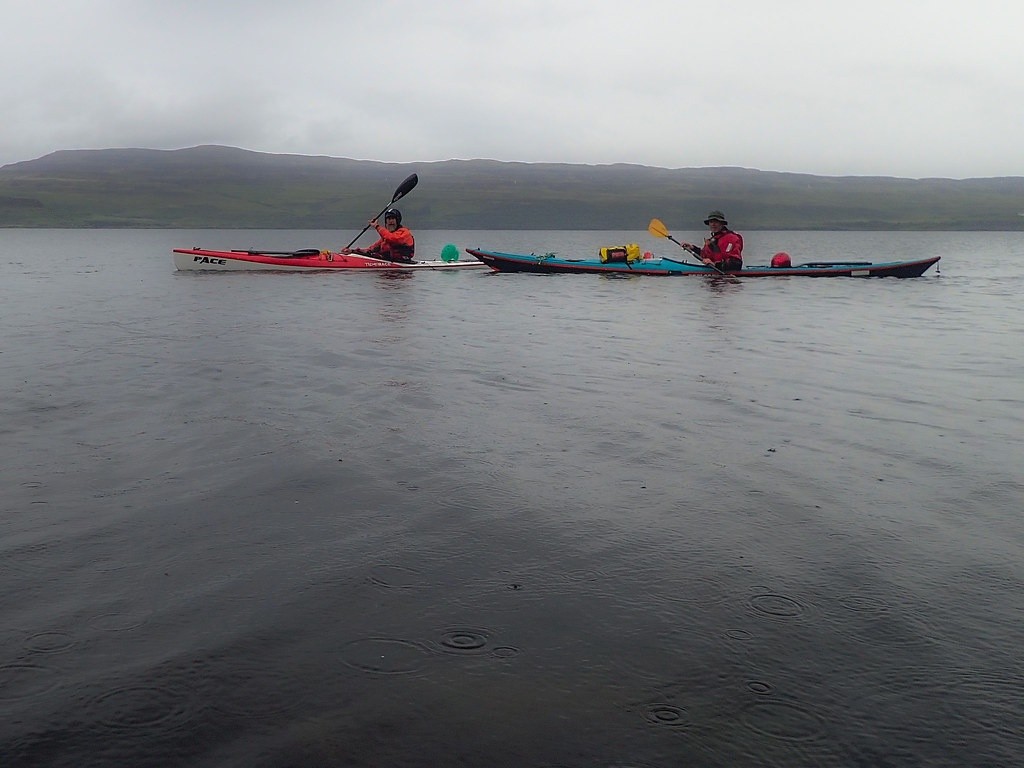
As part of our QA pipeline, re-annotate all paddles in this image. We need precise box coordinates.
[341,172,419,254]
[648,218,741,283]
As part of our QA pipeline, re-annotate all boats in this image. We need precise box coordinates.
[169,248,490,271]
[464,248,941,278]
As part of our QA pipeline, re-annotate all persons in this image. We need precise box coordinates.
[341,209,414,262]
[680,211,744,270]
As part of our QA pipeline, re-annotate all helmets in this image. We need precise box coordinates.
[770,252,791,267]
[385,209,401,226]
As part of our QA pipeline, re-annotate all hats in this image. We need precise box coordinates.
[704,210,728,226]
[385,213,396,219]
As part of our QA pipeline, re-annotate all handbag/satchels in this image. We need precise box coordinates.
[599,244,640,263]
